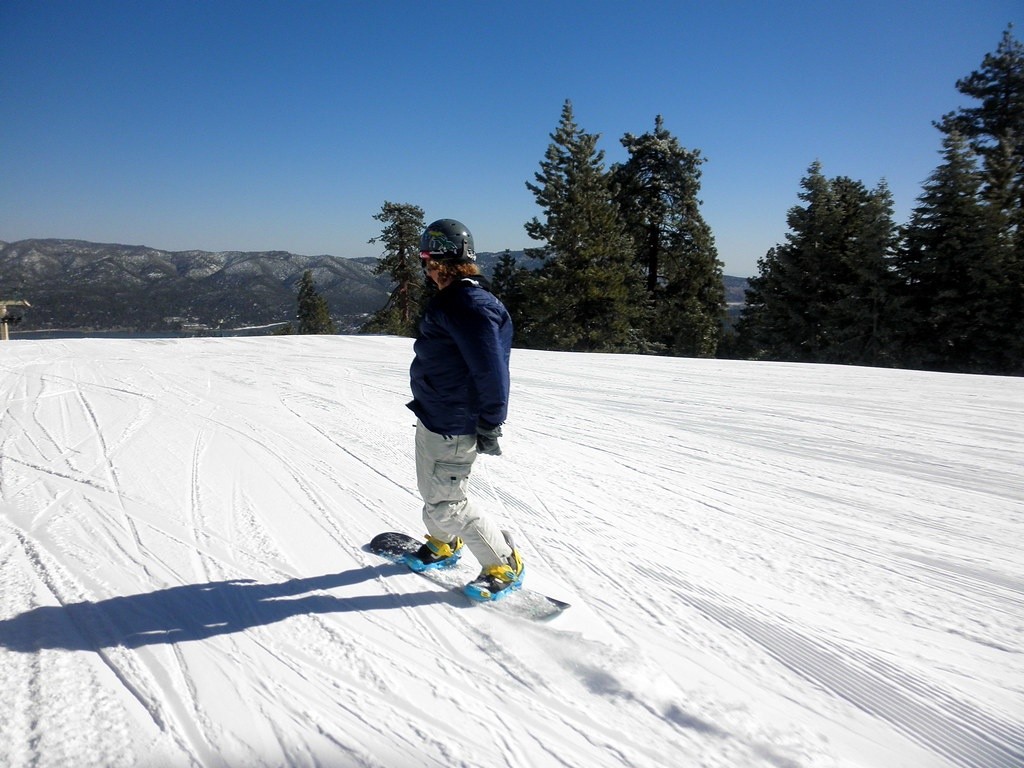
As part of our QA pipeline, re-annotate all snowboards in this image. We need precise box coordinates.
[366,529,572,622]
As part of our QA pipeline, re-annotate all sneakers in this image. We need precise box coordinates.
[405,534,464,572]
[462,530,525,602]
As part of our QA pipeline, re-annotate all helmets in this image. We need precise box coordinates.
[419,219,474,267]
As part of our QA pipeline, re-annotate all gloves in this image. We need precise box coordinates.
[477,426,502,456]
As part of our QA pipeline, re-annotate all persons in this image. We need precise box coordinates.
[406,221,524,600]
